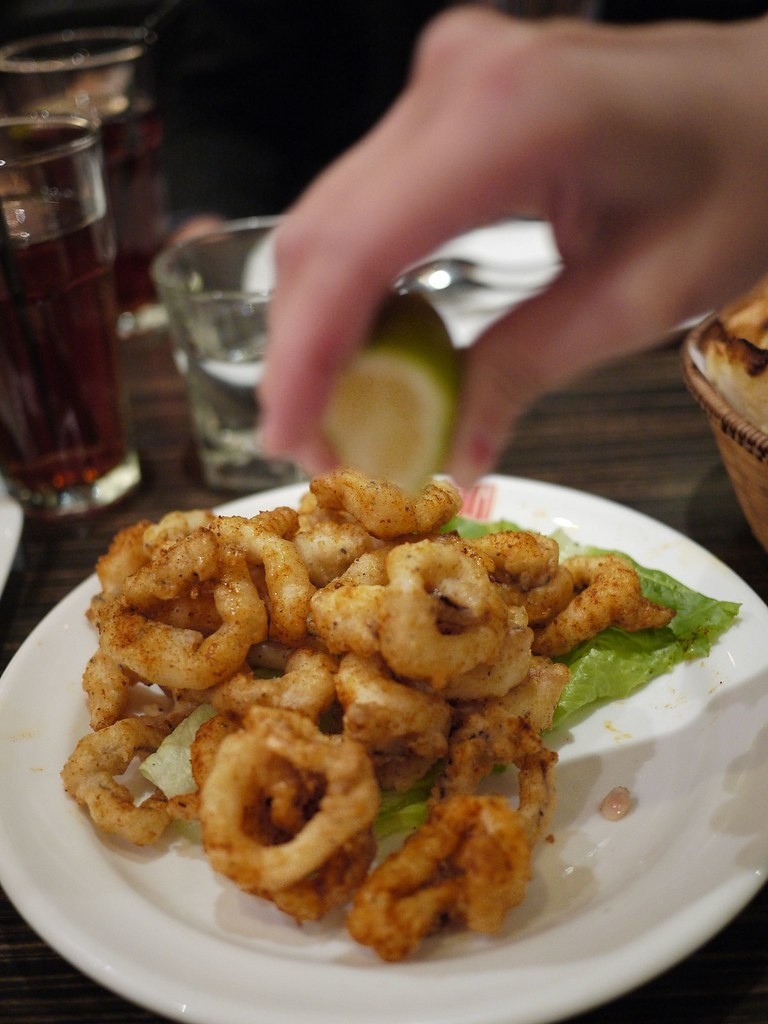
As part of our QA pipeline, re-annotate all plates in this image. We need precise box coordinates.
[242,209,562,353]
[2,471,767,1024]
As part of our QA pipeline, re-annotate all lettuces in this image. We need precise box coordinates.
[356,516,743,835]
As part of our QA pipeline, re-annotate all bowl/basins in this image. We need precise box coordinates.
[681,290,767,551]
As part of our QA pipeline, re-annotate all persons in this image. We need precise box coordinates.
[139,0,581,246]
[256,5,767,491]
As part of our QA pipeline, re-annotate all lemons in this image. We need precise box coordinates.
[313,284,463,492]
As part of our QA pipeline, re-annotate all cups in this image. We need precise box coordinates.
[151,214,308,493]
[0,27,170,336]
[0,113,145,521]
